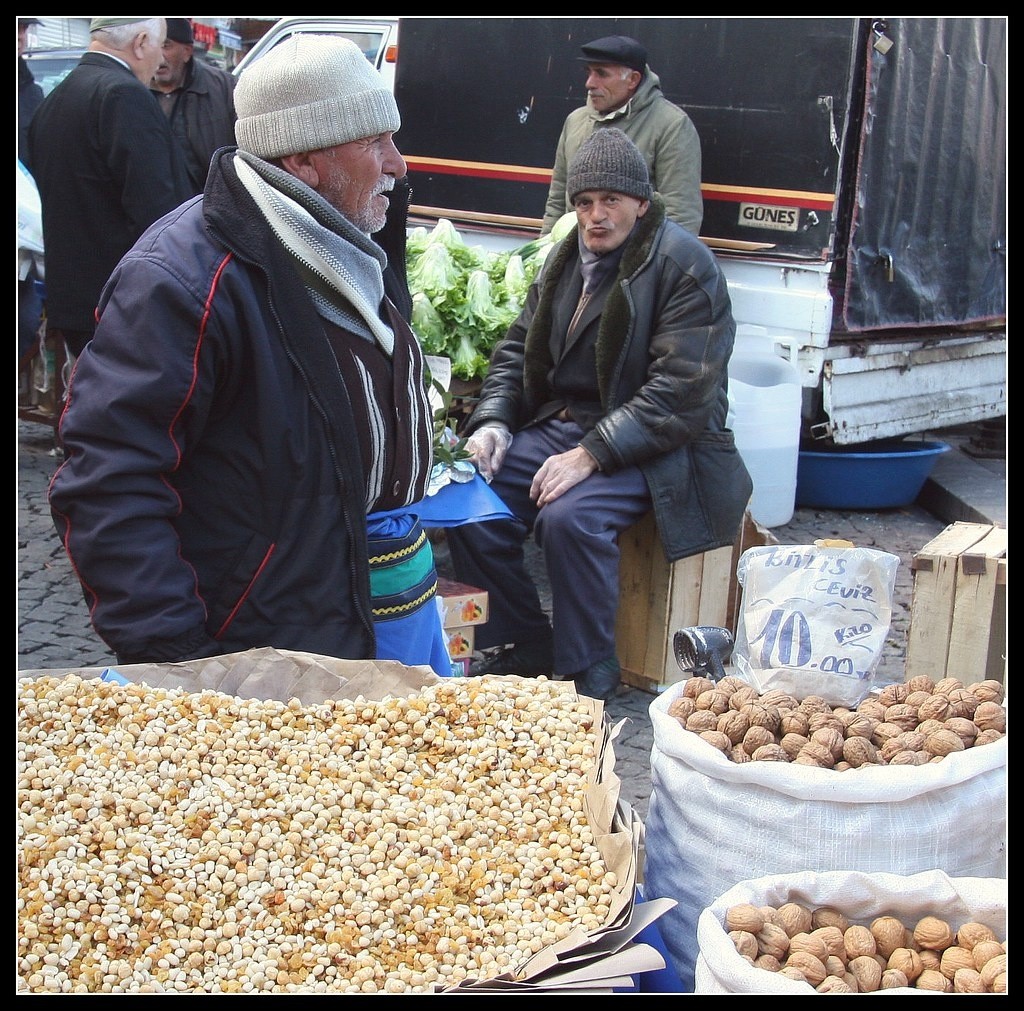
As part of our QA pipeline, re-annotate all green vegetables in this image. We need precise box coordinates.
[406,212,575,380]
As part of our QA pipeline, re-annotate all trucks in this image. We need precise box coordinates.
[233,17,1008,446]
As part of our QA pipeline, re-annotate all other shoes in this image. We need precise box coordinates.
[562,654,621,699]
[468,646,553,680]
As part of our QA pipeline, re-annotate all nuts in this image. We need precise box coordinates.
[723,900,1008,995]
[19,676,616,995]
[667,673,1007,770]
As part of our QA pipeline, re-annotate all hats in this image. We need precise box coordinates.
[577,34,648,73]
[18,18,46,27]
[232,33,402,159]
[165,18,195,44]
[89,18,152,33]
[566,127,652,206]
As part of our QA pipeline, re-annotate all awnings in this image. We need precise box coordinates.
[192,21,242,52]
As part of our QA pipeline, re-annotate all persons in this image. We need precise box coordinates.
[440,118,754,701]
[26,17,194,361]
[16,157,44,361]
[536,34,705,242]
[147,16,241,197]
[47,34,454,686]
[16,17,48,173]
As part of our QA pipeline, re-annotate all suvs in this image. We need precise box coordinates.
[22,47,87,77]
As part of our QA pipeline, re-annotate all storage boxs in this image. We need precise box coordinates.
[450,658,470,677]
[435,576,489,629]
[904,520,1006,686]
[444,626,476,659]
[614,506,734,694]
